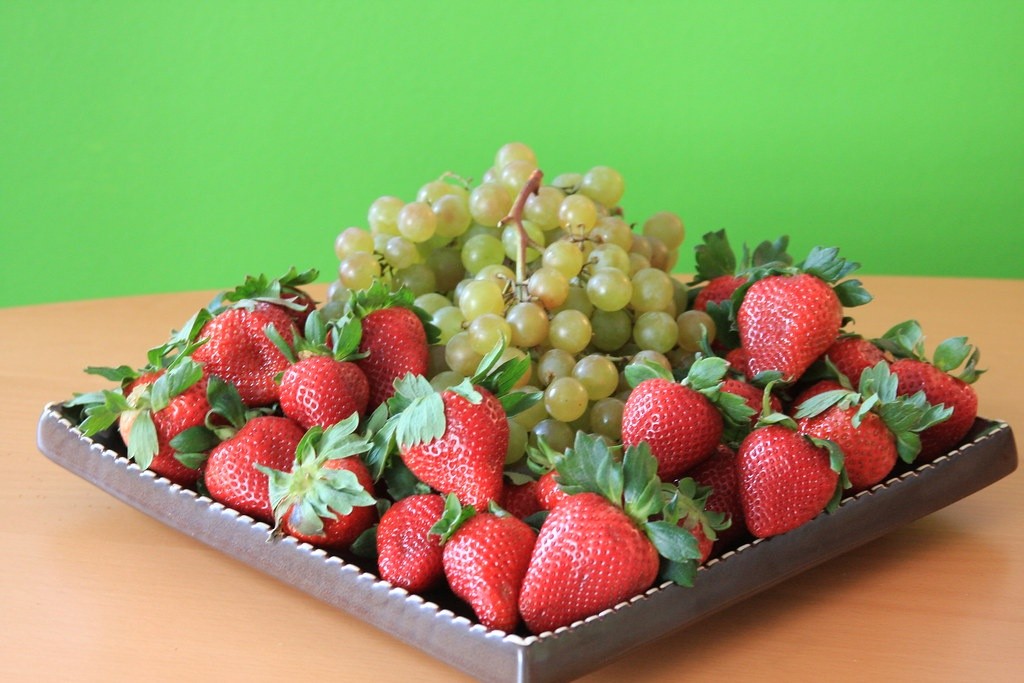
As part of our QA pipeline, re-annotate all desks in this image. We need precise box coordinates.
[0,274,1024,683]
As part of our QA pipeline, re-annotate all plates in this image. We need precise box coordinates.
[39,401,1020,683]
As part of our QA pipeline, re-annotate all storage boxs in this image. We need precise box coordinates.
[35,388,1018,683]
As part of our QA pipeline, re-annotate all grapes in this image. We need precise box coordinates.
[318,139,718,471]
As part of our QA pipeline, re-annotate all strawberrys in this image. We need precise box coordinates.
[61,230,990,634]
